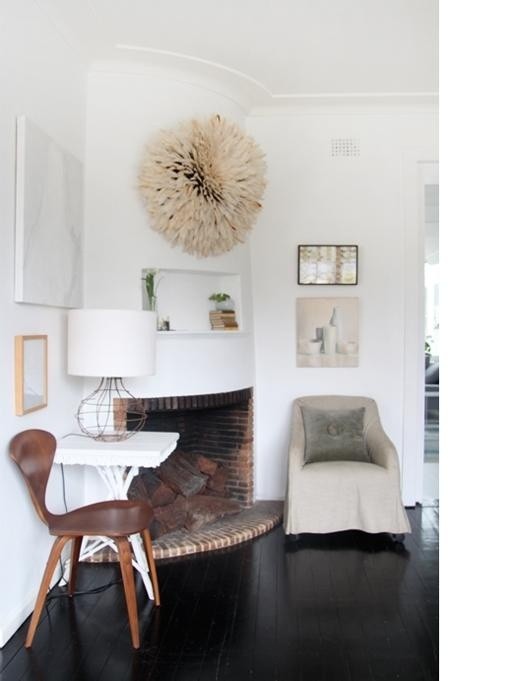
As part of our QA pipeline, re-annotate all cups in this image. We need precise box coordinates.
[321,326,337,355]
[306,341,322,354]
[341,341,356,353]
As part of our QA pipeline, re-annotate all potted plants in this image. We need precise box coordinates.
[207,291,240,331]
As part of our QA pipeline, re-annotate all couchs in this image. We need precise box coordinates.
[426,352,439,419]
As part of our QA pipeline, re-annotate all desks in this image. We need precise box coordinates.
[54,425,181,601]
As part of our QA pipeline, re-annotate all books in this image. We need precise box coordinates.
[209,310,239,331]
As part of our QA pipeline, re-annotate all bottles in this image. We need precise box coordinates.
[330,306,343,341]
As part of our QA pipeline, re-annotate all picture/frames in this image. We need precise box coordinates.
[15,333,48,416]
[297,244,359,286]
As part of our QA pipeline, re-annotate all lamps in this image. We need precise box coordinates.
[66,307,159,444]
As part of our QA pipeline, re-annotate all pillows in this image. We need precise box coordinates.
[426,361,439,384]
[298,403,373,465]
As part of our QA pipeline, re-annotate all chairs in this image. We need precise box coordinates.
[9,429,161,650]
[285,394,412,535]
